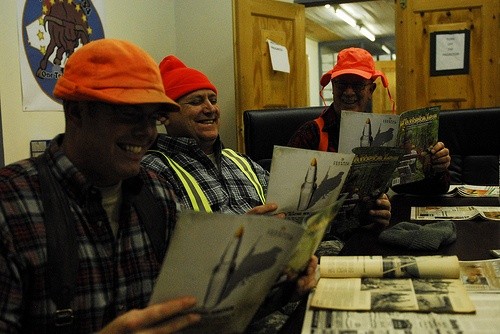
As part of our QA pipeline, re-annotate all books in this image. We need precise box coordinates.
[147,194,349,334]
[338,105,442,186]
[265,144,403,233]
[308,256,476,313]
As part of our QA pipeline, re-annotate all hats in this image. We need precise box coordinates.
[318,48,397,117]
[53,38,182,111]
[160,55,218,101]
[378,221,458,257]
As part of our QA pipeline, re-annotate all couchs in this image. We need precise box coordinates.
[390,107,500,262]
[243,106,327,171]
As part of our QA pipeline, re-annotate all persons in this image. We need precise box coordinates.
[292,47,450,195]
[0,39,317,334]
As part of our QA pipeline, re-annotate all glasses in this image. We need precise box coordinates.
[332,79,373,91]
[102,105,170,126]
